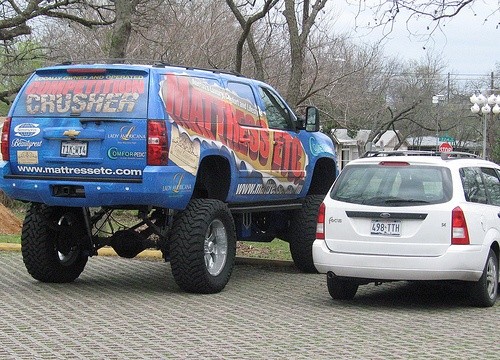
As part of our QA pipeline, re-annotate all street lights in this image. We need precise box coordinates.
[469,93,500,160]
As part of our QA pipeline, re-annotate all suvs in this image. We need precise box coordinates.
[0,58,341,295]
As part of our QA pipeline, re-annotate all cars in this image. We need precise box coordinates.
[312,148,500,307]
